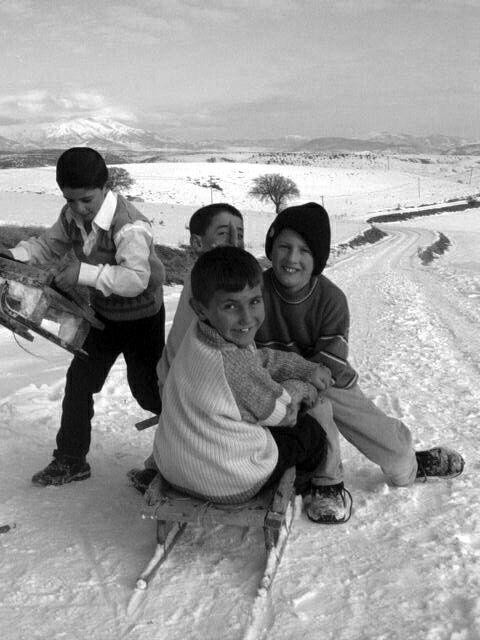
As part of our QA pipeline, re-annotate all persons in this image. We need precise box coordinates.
[0,145,167,491]
[128,244,336,506]
[154,202,246,399]
[253,201,466,525]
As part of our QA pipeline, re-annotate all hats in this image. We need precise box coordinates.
[264,202,331,275]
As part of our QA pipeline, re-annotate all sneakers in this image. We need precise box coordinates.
[415,447,465,478]
[302,483,349,524]
[128,467,157,494]
[33,457,92,487]
